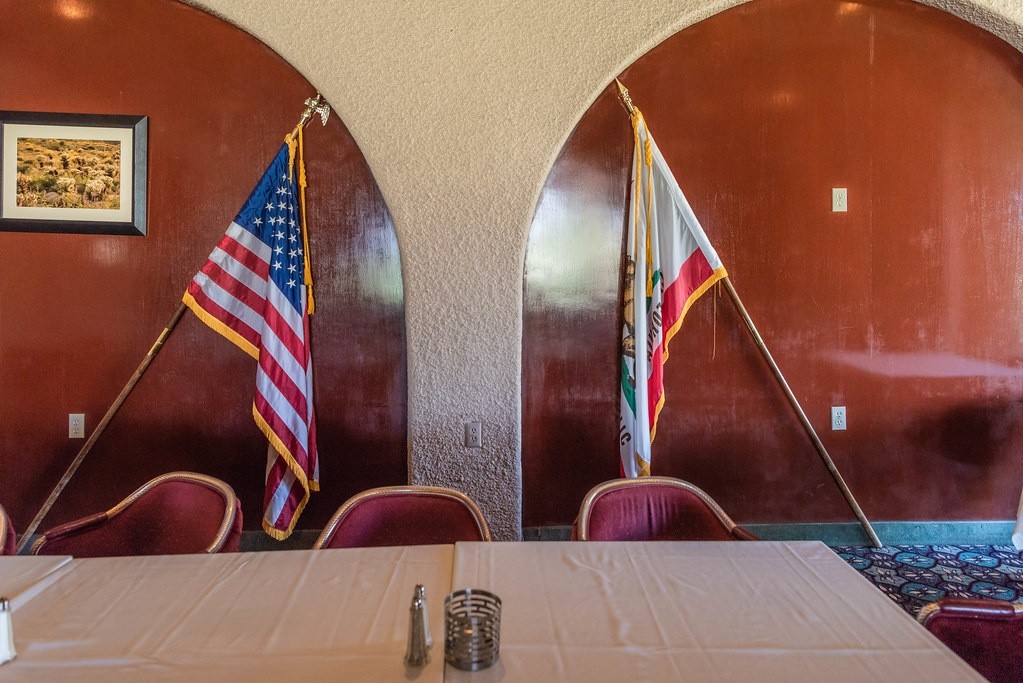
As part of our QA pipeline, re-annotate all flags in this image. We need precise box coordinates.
[179,131,319,542]
[618,109,725,479]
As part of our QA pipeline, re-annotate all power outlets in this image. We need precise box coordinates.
[68,413,84,439]
[830,407,847,430]
[831,188,847,212]
[465,422,482,447]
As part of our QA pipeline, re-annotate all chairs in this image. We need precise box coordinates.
[916,598,1023,683]
[27,471,242,559]
[569,476,761,541]
[313,486,491,550]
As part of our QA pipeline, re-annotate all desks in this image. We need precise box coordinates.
[0,541,994,682]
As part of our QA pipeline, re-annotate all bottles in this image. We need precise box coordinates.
[0,596,17,666]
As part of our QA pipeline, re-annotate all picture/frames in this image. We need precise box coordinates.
[0,110,149,237]
